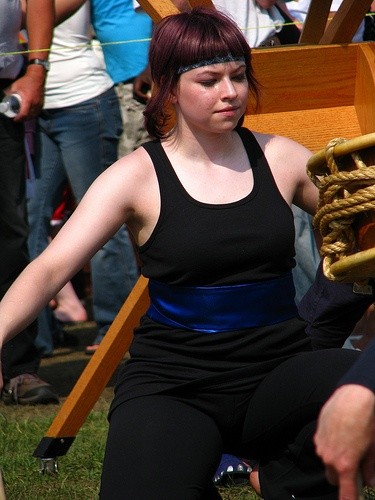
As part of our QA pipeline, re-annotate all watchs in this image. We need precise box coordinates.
[28,58,50,71]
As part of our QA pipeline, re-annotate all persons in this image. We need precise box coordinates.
[0,0,375,500]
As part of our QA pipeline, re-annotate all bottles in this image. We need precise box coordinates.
[0,92,22,118]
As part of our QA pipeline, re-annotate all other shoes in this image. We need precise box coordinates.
[1,373,59,406]
